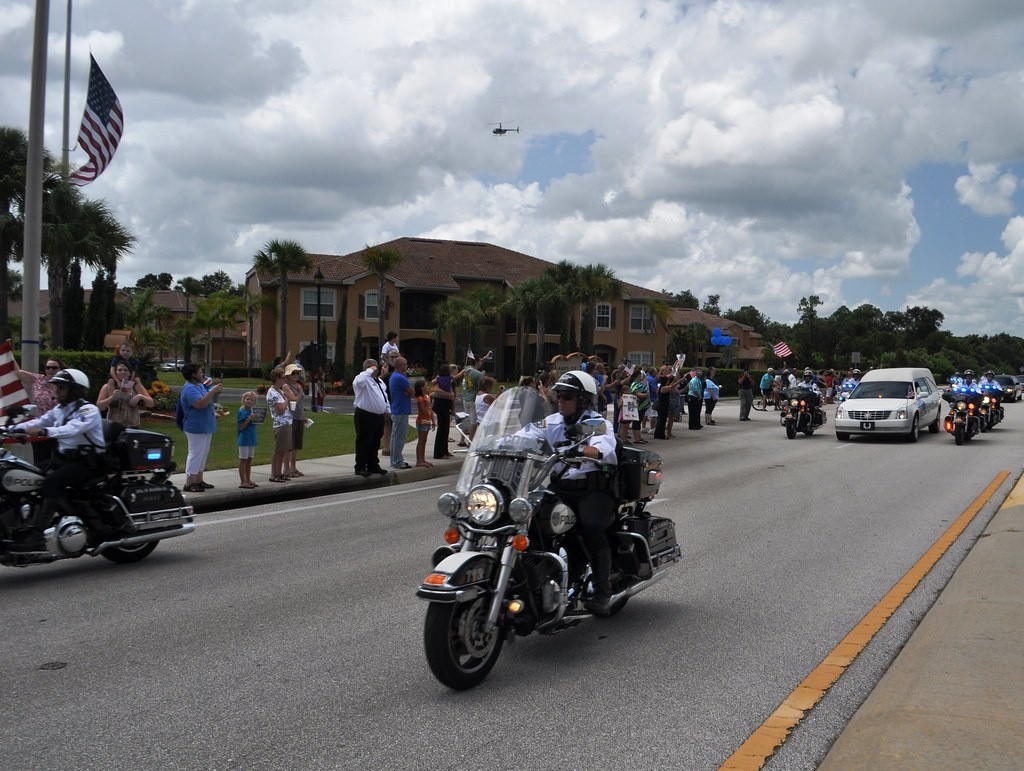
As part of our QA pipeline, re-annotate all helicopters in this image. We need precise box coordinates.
[487,120,520,136]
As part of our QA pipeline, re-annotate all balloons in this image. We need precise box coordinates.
[711,327,732,346]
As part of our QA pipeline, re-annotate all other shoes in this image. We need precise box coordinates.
[382,450,390,456]
[740,417,751,421]
[434,452,454,460]
[690,420,716,432]
[416,461,435,468]
[391,462,412,470]
[616,434,676,447]
[183,481,213,492]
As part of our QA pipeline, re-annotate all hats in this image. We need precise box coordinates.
[284,364,302,375]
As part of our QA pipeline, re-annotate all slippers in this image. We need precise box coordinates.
[238,483,259,489]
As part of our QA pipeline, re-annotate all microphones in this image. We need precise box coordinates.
[51,396,66,400]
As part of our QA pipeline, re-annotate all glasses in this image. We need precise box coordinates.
[46,366,60,370]
[291,371,300,376]
[391,356,398,358]
[400,364,408,366]
[556,395,578,400]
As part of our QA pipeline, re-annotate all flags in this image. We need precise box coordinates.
[467,348,476,361]
[624,362,635,375]
[68,53,123,188]
[836,385,915,398]
[773,341,792,358]
[0,340,51,444]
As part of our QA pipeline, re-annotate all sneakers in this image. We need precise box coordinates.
[366,464,388,475]
[354,464,371,477]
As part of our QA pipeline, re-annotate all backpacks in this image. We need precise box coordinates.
[176,385,204,431]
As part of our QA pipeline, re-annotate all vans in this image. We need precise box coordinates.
[835,367,941,444]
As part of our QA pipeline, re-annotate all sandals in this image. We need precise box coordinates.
[269,474,289,482]
[282,471,305,477]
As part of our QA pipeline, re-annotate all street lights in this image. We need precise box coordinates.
[313,267,324,357]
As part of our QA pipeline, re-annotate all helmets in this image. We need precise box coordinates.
[804,370,813,377]
[48,369,89,391]
[552,371,597,398]
[955,372,961,376]
[853,369,860,374]
[985,371,994,378]
[963,369,974,375]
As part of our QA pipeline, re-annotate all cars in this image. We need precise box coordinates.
[160,360,184,372]
[1016,375,1024,391]
[993,375,1022,403]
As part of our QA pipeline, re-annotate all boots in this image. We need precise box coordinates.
[49,497,95,547]
[585,547,614,616]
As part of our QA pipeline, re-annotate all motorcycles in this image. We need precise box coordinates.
[777,378,827,440]
[942,387,981,445]
[415,386,682,692]
[0,418,196,568]
[979,385,1004,432]
[837,383,855,404]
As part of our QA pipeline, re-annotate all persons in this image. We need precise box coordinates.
[265,349,327,483]
[426,351,505,459]
[496,370,618,612]
[96,343,154,429]
[179,362,224,492]
[6,339,70,467]
[376,331,415,469]
[760,367,874,428]
[352,358,392,477]
[414,380,436,468]
[581,356,720,446]
[946,368,1002,434]
[518,361,559,429]
[738,368,755,421]
[0,369,106,536]
[236,391,259,489]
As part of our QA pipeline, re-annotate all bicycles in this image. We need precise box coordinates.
[751,385,783,410]
